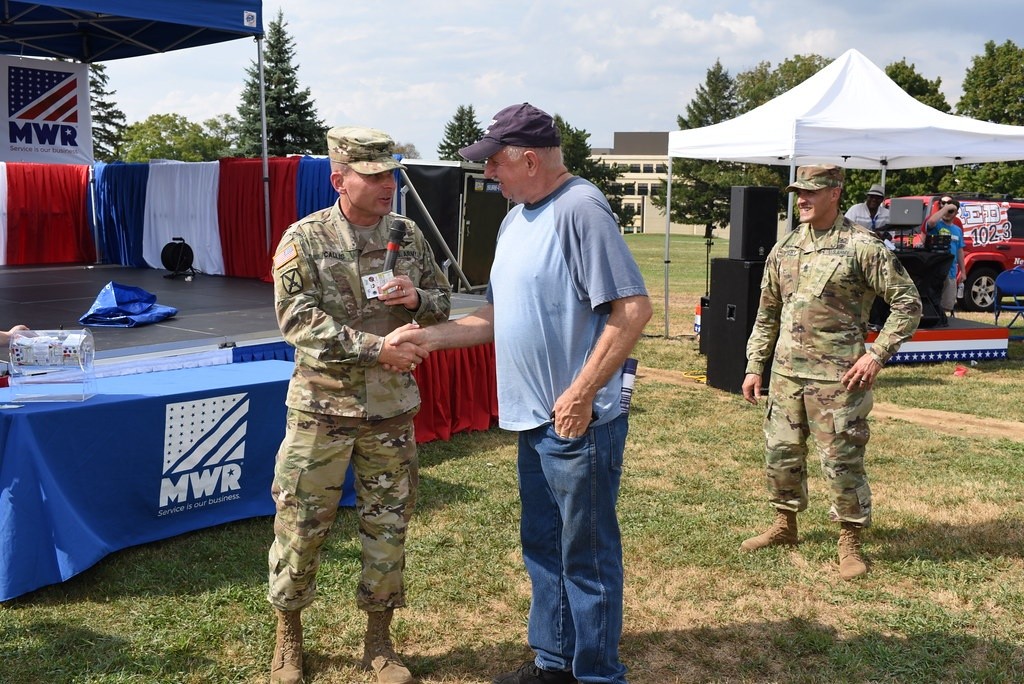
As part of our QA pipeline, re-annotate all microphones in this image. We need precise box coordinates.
[385,219,407,275]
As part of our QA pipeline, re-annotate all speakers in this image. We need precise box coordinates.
[919,294,940,329]
[699,186,780,397]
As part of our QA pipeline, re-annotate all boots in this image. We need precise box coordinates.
[362,609,414,684]
[739,507,799,551]
[837,522,868,581]
[270,610,303,684]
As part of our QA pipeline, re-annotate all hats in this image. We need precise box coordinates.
[458,101,561,161]
[784,165,844,190]
[867,184,883,196]
[327,126,408,174]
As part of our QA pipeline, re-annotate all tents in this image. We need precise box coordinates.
[0,0,271,287]
[662,48,1024,339]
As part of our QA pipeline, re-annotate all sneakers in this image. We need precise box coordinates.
[490,655,578,684]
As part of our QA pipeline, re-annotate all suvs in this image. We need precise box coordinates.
[880,196,1024,312]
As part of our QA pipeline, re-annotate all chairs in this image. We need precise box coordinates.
[993,265,1024,341]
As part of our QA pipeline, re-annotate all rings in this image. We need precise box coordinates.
[860,379,868,382]
[402,289,405,297]
[408,361,418,371]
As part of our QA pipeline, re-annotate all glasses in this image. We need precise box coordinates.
[948,210,954,214]
[941,201,948,205]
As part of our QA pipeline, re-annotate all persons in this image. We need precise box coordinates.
[921,196,966,315]
[0,324,31,348]
[844,184,895,241]
[741,165,923,580]
[266,127,452,684]
[383,102,651,684]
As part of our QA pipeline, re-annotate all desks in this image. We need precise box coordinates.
[0,360,356,602]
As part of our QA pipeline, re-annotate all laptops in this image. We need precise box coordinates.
[876,199,923,231]
